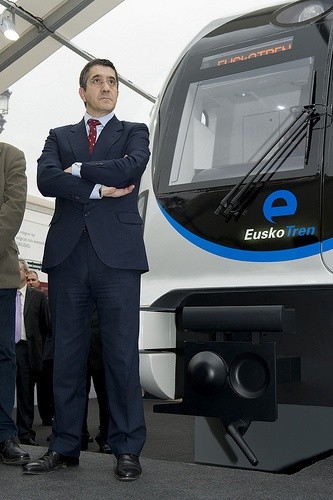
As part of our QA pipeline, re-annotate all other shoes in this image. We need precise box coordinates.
[80,444,88,451]
[43,421,54,425]
[94,445,112,454]
[21,439,41,446]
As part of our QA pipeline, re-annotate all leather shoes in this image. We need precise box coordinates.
[114,454,142,481]
[0,438,31,465]
[23,450,79,474]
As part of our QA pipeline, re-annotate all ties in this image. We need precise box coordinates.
[15,291,22,343]
[87,118,101,158]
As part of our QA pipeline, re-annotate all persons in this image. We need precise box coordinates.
[15,259,114,453]
[0,141,31,464]
[22,59,151,481]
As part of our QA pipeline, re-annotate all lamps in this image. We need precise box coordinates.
[0,7,19,41]
